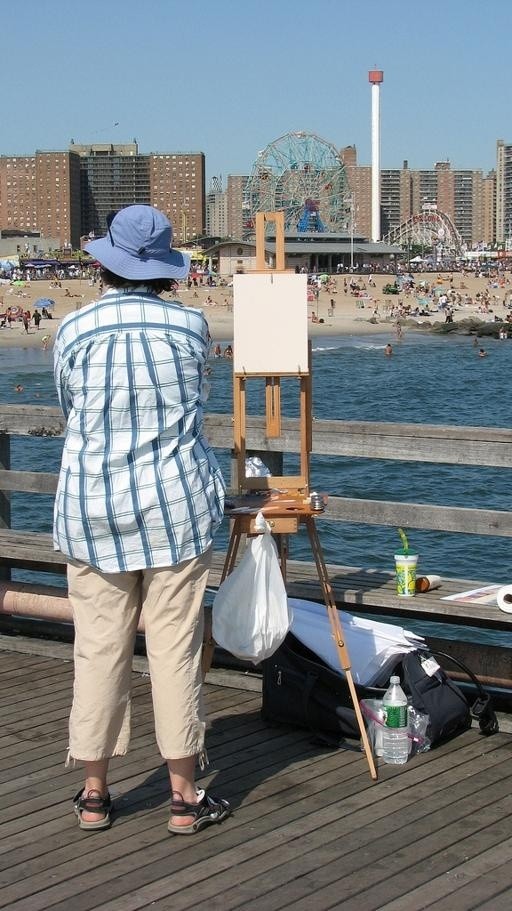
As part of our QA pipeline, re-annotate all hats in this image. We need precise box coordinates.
[81,205,191,280]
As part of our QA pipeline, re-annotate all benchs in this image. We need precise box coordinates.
[0,406,510,628]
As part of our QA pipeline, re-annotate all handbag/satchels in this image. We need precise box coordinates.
[261,632,472,751]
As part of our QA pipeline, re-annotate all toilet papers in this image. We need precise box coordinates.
[497,584,512,614]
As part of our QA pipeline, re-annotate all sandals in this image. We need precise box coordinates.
[167,785,231,834]
[73,786,113,830]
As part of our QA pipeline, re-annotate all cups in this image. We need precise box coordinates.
[392,545,424,598]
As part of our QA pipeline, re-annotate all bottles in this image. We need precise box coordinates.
[383,672,410,763]
[415,573,441,591]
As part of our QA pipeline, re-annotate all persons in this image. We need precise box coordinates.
[36,203,235,835]
[1,238,509,394]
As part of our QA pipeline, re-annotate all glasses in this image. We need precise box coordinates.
[106,213,118,247]
